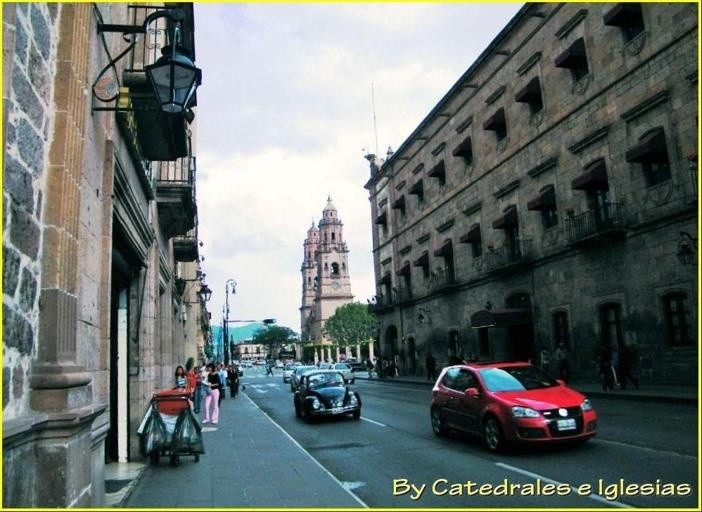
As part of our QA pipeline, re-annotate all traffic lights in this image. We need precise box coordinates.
[263,318,276,324]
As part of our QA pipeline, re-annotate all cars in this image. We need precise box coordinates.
[235,363,243,377]
[282,358,362,423]
[431,360,597,453]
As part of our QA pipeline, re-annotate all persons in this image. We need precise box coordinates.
[266,363,274,375]
[424,351,437,381]
[174,355,240,425]
[597,340,617,389]
[553,339,572,382]
[366,357,397,379]
[537,344,553,372]
[615,336,640,390]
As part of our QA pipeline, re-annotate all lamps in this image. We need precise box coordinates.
[91,10,202,116]
[484,301,496,311]
[417,308,432,324]
[676,231,698,265]
[182,278,212,303]
[173,277,187,296]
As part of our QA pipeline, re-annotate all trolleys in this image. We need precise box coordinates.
[136,388,205,466]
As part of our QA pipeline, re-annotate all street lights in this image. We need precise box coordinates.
[225,278,237,364]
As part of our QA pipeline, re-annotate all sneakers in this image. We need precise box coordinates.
[201,419,218,424]
